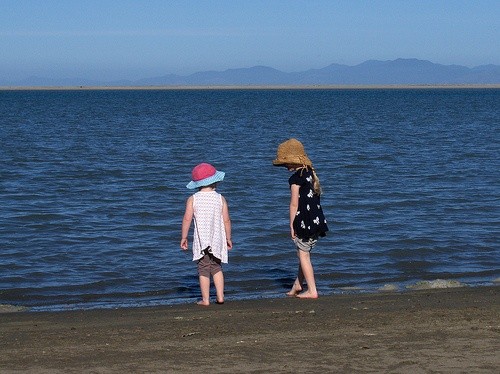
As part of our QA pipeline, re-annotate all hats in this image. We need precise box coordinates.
[273,137,313,167]
[186,162,226,189]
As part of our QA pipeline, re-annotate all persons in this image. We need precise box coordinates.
[273,138,329,298]
[179,162,234,307]
